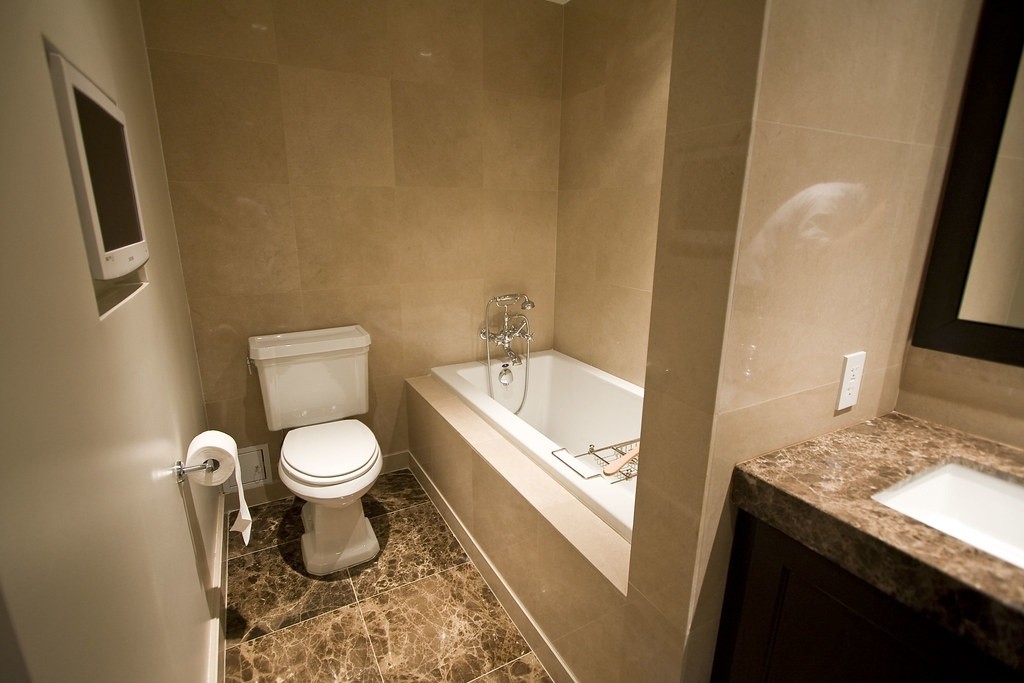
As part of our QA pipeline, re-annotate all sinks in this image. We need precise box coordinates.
[871,451,1023,584]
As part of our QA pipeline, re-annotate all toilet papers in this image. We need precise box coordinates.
[184,430,253,548]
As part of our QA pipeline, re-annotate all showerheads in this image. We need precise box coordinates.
[492,293,536,311]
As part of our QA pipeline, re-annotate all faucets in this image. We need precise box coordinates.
[501,345,524,367]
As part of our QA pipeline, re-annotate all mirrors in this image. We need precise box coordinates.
[909,0,1024,366]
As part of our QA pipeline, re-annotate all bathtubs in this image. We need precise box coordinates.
[404,348,645,683]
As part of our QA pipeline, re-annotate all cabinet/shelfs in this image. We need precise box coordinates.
[727,518,1024,683]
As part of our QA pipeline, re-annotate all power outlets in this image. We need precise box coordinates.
[835,351,866,411]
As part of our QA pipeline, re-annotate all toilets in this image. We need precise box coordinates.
[246,322,386,579]
